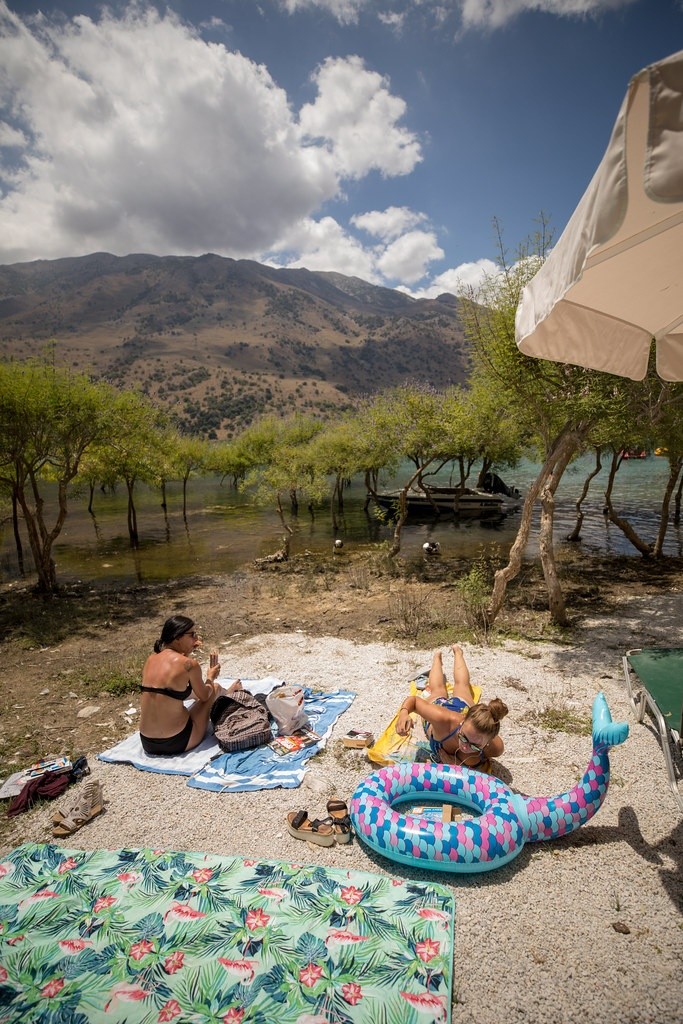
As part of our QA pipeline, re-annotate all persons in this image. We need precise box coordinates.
[271,731,313,756]
[396,644,509,770]
[139,614,242,756]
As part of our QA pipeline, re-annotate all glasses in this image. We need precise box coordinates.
[185,631,198,640]
[458,732,487,752]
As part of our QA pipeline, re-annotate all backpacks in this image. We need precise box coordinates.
[209,689,271,753]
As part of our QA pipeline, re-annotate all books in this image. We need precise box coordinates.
[24,755,72,782]
[265,725,322,756]
[343,728,375,748]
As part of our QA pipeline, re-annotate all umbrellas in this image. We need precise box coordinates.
[515,49,683,382]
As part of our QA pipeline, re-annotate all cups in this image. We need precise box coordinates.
[210,649,218,668]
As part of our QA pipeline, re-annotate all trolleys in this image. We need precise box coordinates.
[624,646,683,817]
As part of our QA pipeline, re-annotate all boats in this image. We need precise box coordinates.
[366,486,524,519]
[620,448,646,460]
[654,447,676,455]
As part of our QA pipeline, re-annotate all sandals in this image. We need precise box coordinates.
[52,778,105,836]
[286,810,335,847]
[327,797,350,843]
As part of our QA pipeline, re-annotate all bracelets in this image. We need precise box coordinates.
[400,708,410,715]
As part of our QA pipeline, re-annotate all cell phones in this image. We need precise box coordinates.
[405,719,413,731]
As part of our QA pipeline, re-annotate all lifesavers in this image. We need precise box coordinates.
[349,689,629,873]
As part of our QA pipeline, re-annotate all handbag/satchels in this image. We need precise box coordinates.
[265,686,308,736]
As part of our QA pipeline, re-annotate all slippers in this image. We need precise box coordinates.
[407,665,432,690]
